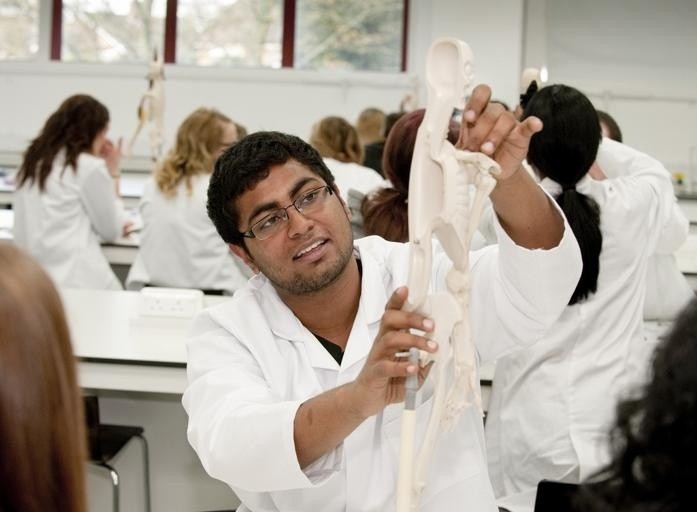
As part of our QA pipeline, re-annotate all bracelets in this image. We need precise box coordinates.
[111,173,122,179]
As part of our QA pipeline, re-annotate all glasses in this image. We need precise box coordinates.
[239,185,334,242]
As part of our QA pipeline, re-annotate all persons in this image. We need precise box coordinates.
[7,93,128,291]
[0,237,97,510]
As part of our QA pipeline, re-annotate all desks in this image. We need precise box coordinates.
[1,172,242,393]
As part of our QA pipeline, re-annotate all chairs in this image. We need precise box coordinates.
[82,395,152,512]
